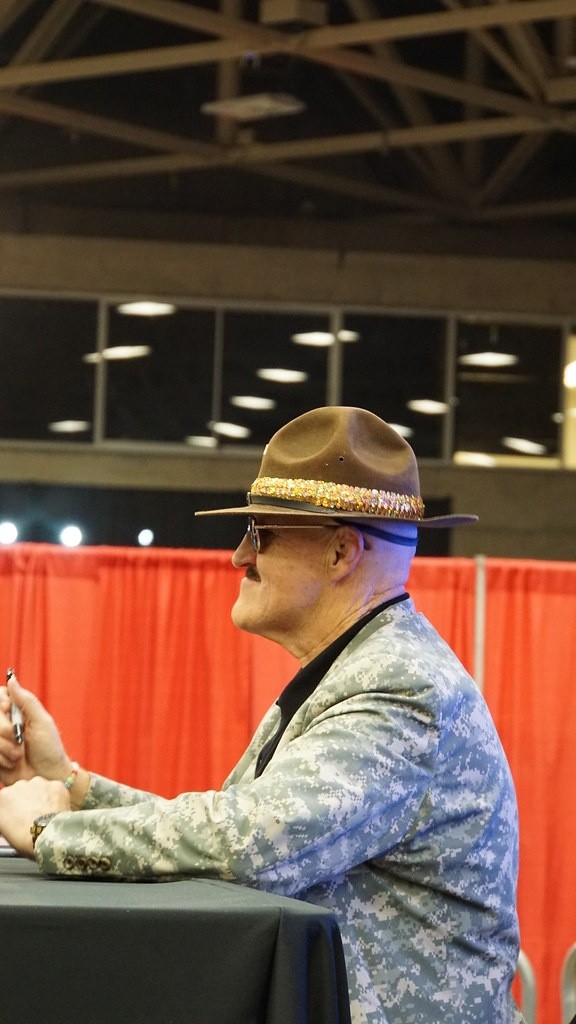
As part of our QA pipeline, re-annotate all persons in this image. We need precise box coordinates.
[1,407,526,1024]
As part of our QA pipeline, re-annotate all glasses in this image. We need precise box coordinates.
[246,514,372,554]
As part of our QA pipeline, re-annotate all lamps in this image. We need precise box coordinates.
[259,0,327,34]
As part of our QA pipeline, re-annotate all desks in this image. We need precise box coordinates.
[0,855,351,1024]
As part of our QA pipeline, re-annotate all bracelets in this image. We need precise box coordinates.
[64,761,80,789]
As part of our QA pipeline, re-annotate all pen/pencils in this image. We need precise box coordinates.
[6,668,23,745]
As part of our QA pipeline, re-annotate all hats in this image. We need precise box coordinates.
[194,406,478,537]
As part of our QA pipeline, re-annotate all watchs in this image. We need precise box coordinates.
[29,812,61,849]
